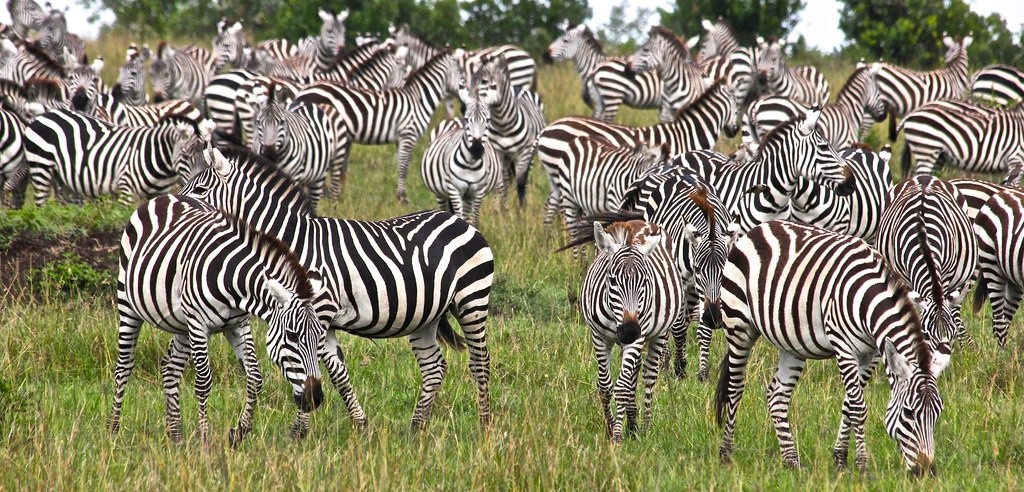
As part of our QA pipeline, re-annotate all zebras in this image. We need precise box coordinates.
[0,8,548,447]
[515,19,1024,470]
